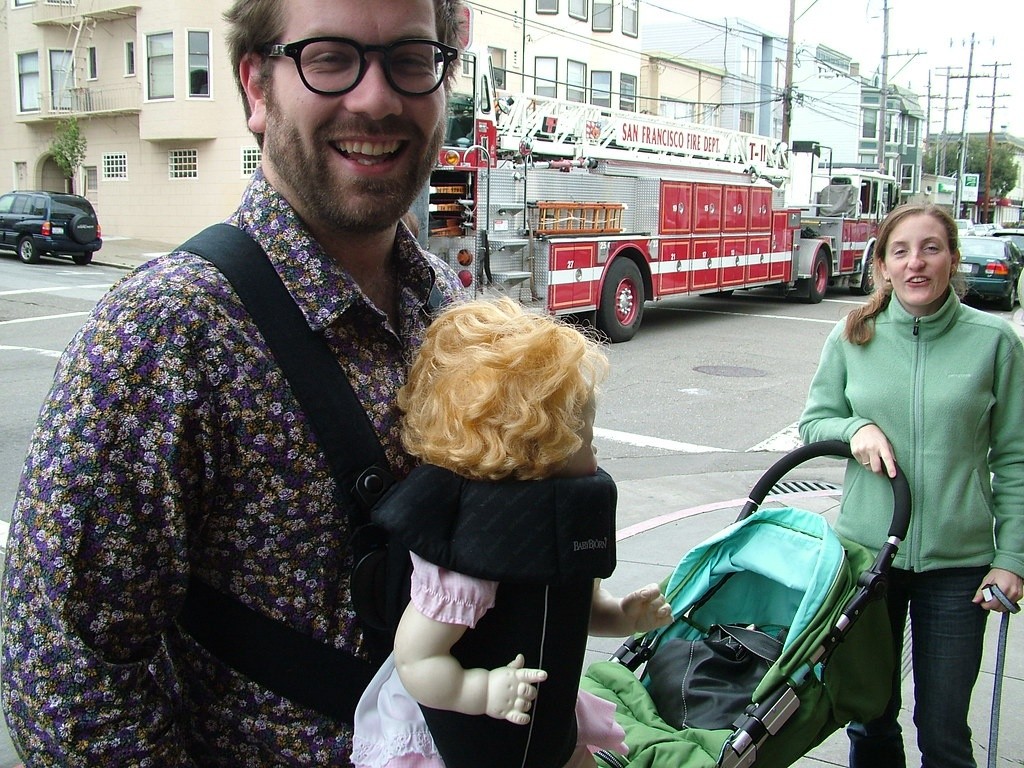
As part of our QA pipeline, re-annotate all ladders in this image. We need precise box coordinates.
[536,200,629,235]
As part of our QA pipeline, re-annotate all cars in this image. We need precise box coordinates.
[953,216,976,237]
[975,221,1003,237]
[951,236,1024,312]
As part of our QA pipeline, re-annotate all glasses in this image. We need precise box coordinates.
[262,35,457,95]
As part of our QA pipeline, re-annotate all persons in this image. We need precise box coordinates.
[350,295,673,768]
[799,205,1024,768]
[1,2,468,768]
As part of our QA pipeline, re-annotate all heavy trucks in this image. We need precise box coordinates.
[418,40,897,345]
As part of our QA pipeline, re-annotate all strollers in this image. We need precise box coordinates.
[558,438,912,768]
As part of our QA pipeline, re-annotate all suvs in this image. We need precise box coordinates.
[0,189,103,266]
